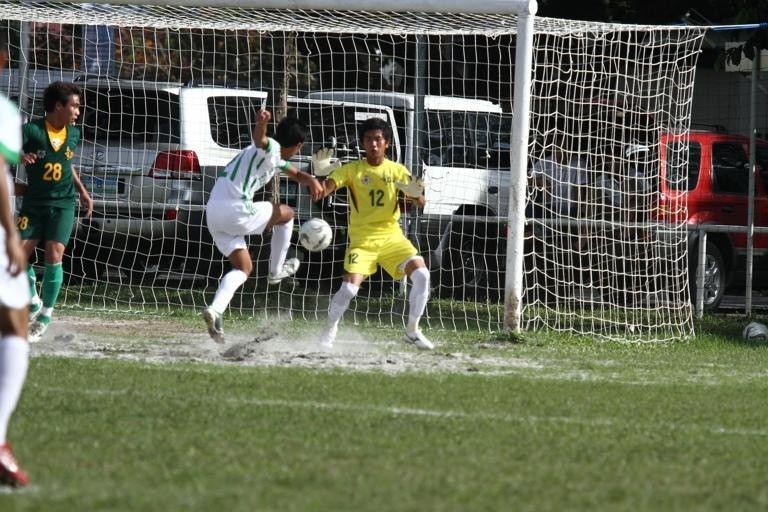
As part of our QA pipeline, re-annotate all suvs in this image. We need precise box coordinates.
[281,89,537,298]
[72,74,300,277]
[0,67,110,221]
[550,99,768,313]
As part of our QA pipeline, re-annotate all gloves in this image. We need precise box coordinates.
[310,145,341,179]
[395,175,424,198]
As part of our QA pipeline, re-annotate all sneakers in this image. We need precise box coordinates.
[316,321,337,351]
[202,307,226,345]
[266,256,301,285]
[403,329,434,350]
[0,443,28,488]
[25,299,50,343]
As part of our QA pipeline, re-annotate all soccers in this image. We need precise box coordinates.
[299,218,332,252]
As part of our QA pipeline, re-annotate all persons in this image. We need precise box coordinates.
[203,110,323,343]
[0,27,32,490]
[318,118,432,350]
[15,81,93,341]
